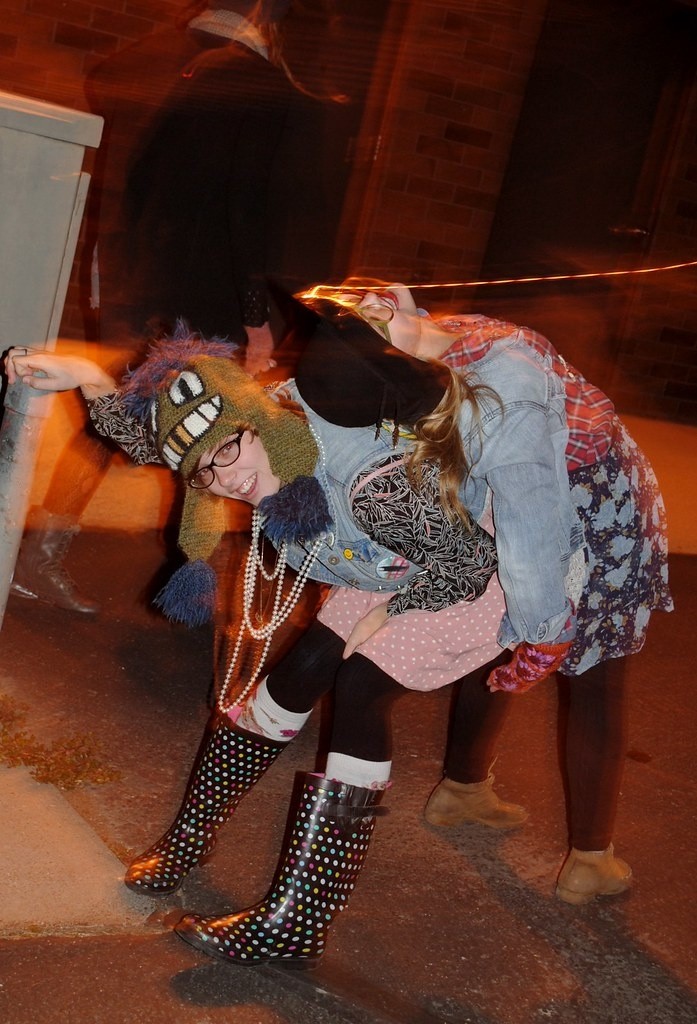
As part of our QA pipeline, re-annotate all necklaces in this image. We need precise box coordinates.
[218,420,327,713]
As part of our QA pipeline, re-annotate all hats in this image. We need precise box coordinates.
[124,318,328,625]
[296,308,450,446]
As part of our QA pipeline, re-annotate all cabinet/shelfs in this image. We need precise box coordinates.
[0,87,105,632]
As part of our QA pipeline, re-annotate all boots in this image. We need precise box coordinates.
[125,710,292,895]
[176,770,393,973]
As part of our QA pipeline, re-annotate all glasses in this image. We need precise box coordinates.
[312,293,394,345]
[186,430,244,490]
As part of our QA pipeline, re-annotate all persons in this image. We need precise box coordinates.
[3,319,514,971]
[276,277,676,904]
[11,0,351,616]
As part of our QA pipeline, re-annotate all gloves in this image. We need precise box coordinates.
[486,643,572,695]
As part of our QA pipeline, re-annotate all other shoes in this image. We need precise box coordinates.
[423,755,529,830]
[556,842,632,906]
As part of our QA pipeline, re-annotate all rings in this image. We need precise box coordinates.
[24,348,27,355]
